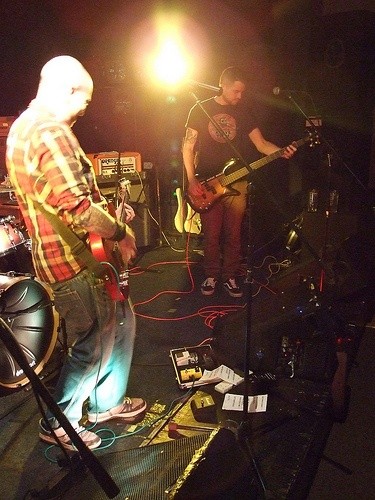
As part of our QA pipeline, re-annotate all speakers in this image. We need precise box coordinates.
[124,184,156,249]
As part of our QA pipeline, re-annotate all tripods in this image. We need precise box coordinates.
[168,94,325,500]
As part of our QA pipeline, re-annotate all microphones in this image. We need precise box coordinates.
[272,87,309,95]
[191,80,224,97]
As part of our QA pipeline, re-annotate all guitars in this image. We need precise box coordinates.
[89,176,132,302]
[185,129,321,214]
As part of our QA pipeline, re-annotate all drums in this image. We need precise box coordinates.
[0,271,60,389]
[0,213,26,259]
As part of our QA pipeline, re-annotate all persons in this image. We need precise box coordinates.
[182,67,298,299]
[5,55,148,452]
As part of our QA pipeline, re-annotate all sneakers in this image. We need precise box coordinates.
[39,418,102,451]
[223,277,243,297]
[200,277,217,295]
[88,397,147,423]
